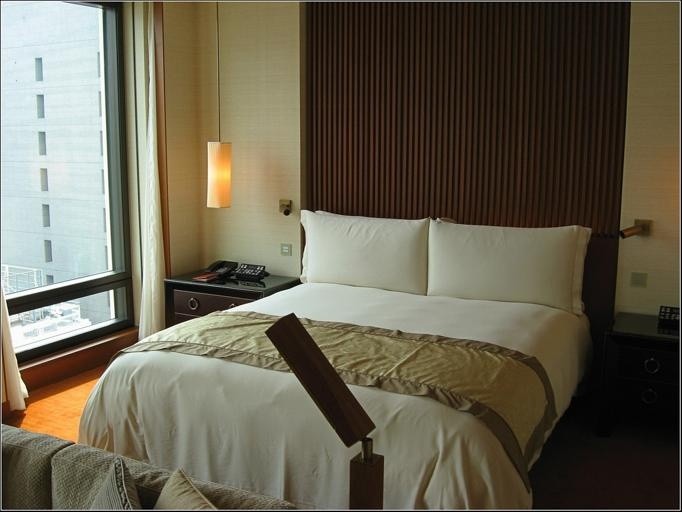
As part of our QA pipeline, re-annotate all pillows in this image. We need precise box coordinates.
[299,208,592,316]
[150,465,223,512]
[88,455,144,511]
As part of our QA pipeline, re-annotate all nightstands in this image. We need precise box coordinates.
[612,312,680,429]
[163,266,302,328]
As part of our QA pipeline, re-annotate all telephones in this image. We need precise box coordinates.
[205,260,238,276]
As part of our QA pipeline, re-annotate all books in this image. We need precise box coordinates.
[193,271,220,283]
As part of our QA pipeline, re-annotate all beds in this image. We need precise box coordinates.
[79,283,593,512]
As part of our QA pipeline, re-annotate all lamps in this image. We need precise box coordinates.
[277,199,292,217]
[620,218,654,240]
[206,2,234,209]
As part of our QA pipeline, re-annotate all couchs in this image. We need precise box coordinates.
[2,421,301,512]
